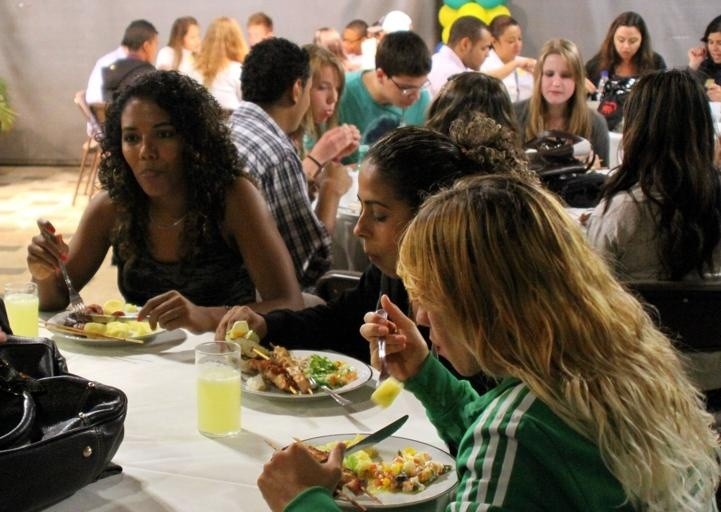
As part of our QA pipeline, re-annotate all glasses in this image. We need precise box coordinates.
[389,75,432,97]
[342,37,363,46]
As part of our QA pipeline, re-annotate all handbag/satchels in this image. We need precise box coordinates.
[599,76,637,127]
[520,127,599,194]
[0,336,129,509]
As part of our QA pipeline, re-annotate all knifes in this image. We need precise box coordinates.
[320,414,408,464]
[68,312,150,324]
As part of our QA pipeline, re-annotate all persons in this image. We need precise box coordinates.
[257,173,721,512]
[0,297,12,343]
[27,9,720,392]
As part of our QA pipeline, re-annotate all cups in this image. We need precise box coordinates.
[3,281,40,338]
[195,341,244,439]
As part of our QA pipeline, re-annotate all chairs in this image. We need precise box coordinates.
[69,88,94,207]
[614,274,719,423]
[85,102,110,207]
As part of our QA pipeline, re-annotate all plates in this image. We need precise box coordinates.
[277,433,462,507]
[47,306,168,347]
[244,351,373,402]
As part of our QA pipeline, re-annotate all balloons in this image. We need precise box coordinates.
[431,0,513,52]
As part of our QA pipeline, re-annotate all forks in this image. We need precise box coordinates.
[306,373,353,409]
[377,296,395,387]
[49,236,88,314]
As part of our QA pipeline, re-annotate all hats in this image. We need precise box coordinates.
[365,9,414,35]
